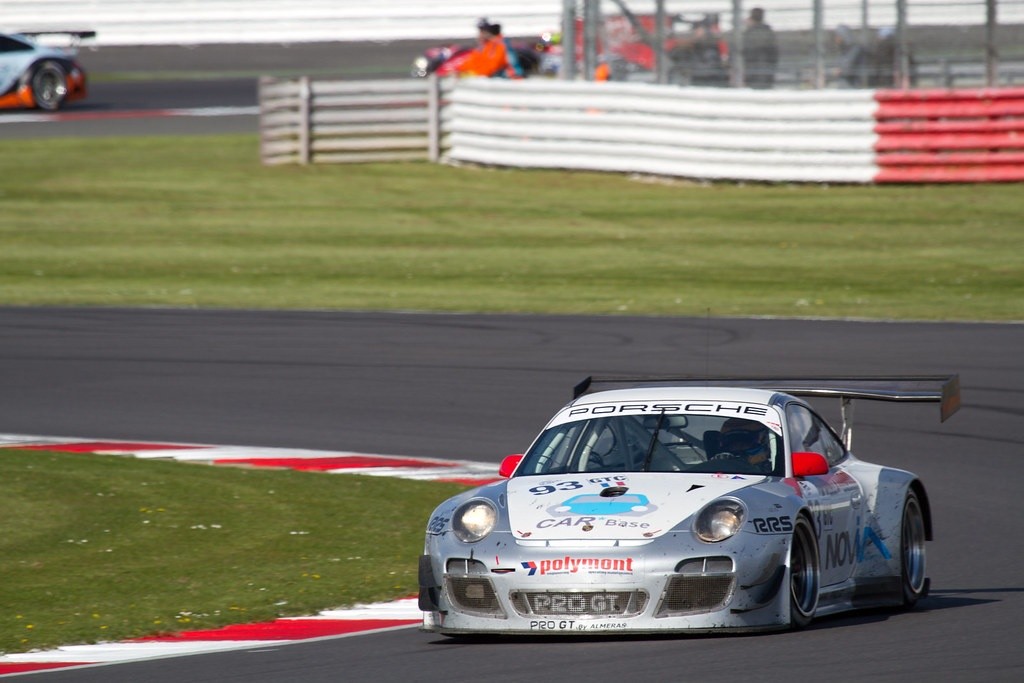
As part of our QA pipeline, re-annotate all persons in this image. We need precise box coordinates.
[669,13,731,87]
[742,8,777,88]
[712,417,777,471]
[451,17,525,79]
[823,24,918,88]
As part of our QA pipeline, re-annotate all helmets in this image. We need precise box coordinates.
[721,418,770,464]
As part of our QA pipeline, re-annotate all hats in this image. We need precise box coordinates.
[478,18,501,34]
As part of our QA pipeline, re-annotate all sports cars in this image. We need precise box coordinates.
[0,26,99,112]
[416,367,963,638]
